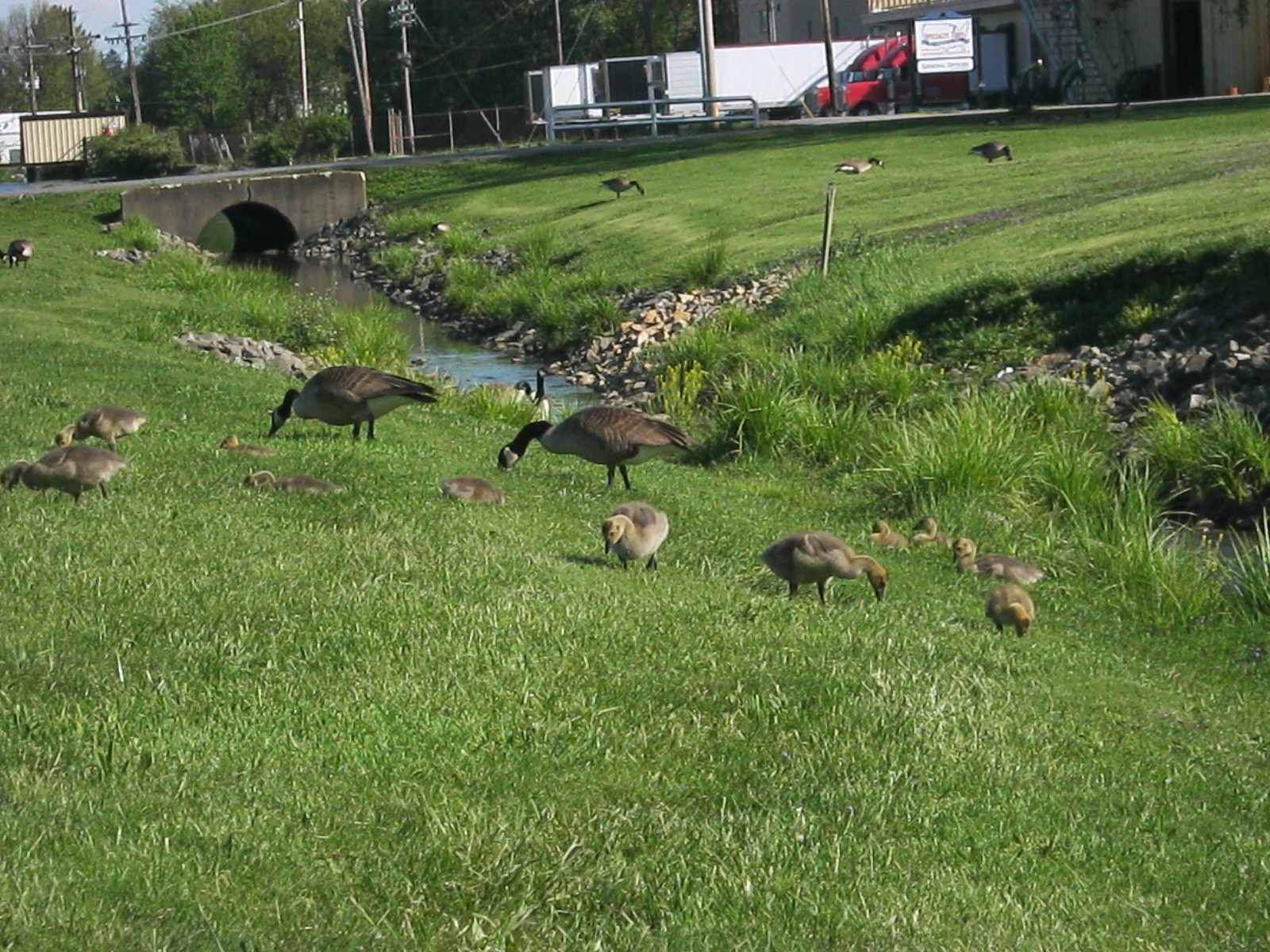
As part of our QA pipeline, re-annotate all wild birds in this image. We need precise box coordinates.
[0,238,33,270]
[498,404,696,490]
[245,471,345,494]
[954,538,1050,586]
[515,366,550,420]
[601,178,646,197]
[868,515,956,554]
[760,530,887,605]
[57,406,148,455]
[266,363,449,440]
[220,434,279,458]
[985,582,1035,637]
[602,499,670,572]
[968,141,1013,162]
[440,476,507,506]
[833,155,883,175]
[0,446,131,502]
[429,222,450,237]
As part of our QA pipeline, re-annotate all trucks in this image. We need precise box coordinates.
[810,32,968,114]
